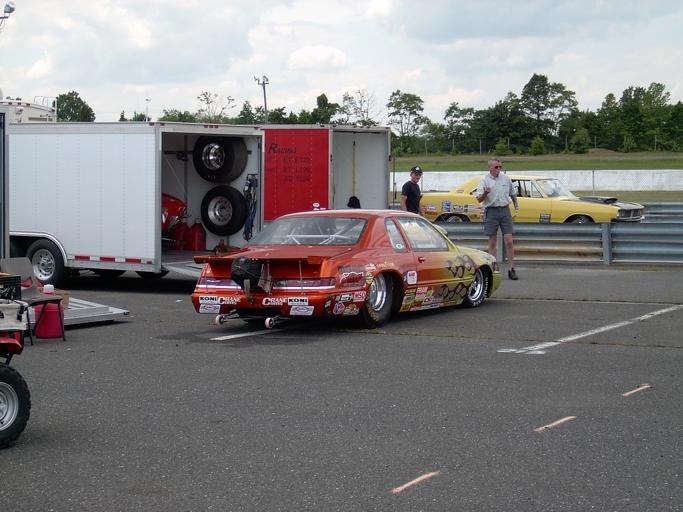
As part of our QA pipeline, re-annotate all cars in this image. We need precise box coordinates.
[408,172,647,229]
[188,209,502,330]
[160,190,189,251]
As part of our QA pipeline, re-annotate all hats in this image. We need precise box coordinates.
[410,165,423,174]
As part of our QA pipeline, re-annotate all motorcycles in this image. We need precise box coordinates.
[0,271,32,448]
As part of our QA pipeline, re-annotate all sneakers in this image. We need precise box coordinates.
[507,269,519,280]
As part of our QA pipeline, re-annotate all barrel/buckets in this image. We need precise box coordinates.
[187,217,206,250]
[173,218,188,251]
[34,285,65,339]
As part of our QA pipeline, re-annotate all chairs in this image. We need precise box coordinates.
[0,257,66,344]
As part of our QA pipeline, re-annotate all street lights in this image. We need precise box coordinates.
[254,75,270,123]
[143,96,152,122]
[0,2,15,33]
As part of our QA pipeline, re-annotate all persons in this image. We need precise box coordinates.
[474,157,519,282]
[399,165,425,217]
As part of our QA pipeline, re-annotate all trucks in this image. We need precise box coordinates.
[1,95,264,293]
[253,123,393,243]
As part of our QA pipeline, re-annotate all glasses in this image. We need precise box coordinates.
[412,173,421,176]
[491,165,502,170]
